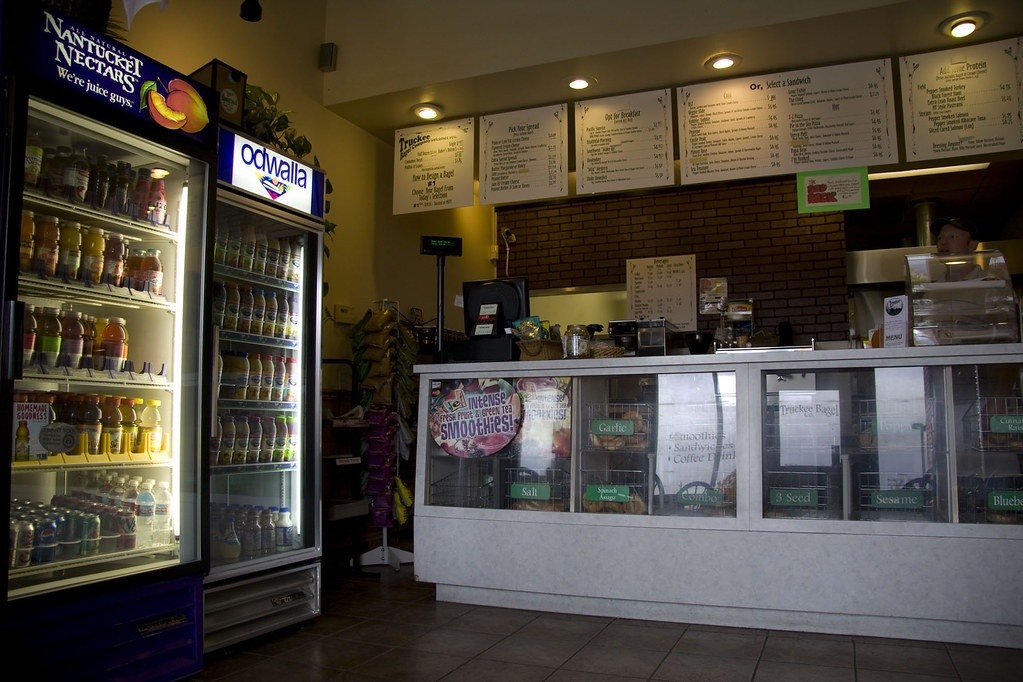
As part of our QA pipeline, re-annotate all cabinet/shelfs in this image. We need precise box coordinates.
[320,357,381,582]
[412,342,1023,651]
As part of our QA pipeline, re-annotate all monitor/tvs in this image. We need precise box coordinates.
[462,275,530,336]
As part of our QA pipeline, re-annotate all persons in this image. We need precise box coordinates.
[920,216,1012,325]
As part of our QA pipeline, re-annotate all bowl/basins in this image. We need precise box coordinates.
[683,332,714,355]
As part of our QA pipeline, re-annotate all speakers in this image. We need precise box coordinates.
[318,42,336,73]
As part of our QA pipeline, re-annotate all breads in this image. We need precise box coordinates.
[588,410,649,451]
[512,494,649,515]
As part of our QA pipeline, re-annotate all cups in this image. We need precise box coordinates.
[736,336,748,348]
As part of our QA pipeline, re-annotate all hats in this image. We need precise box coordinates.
[930,214,979,240]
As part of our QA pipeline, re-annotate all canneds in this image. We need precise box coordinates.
[9,494,136,570]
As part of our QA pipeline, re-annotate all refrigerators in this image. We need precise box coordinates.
[0,0,209,682]
[204,123,326,658]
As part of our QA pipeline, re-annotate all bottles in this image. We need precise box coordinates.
[10,123,170,546]
[411,325,466,346]
[562,324,590,359]
[211,218,301,561]
